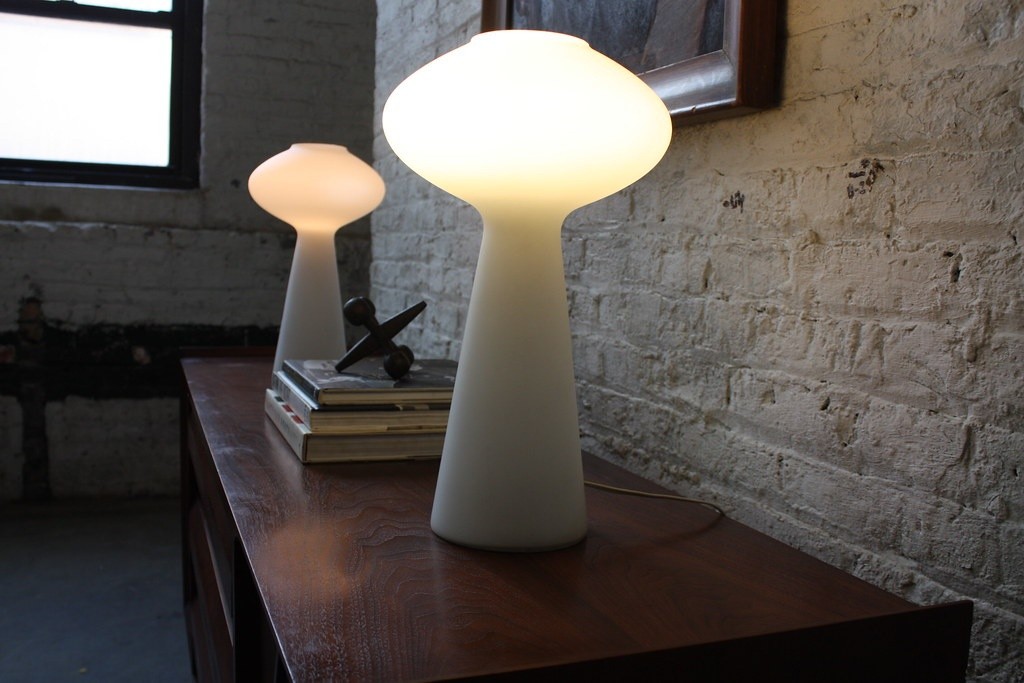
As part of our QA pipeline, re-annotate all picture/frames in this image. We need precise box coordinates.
[481,1,788,128]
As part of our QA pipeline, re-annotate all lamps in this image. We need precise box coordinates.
[381,30,726,554]
[247,144,386,373]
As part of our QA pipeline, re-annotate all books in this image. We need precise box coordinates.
[265,358,458,463]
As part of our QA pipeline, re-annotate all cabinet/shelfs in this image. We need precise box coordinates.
[180,353,974,683]
